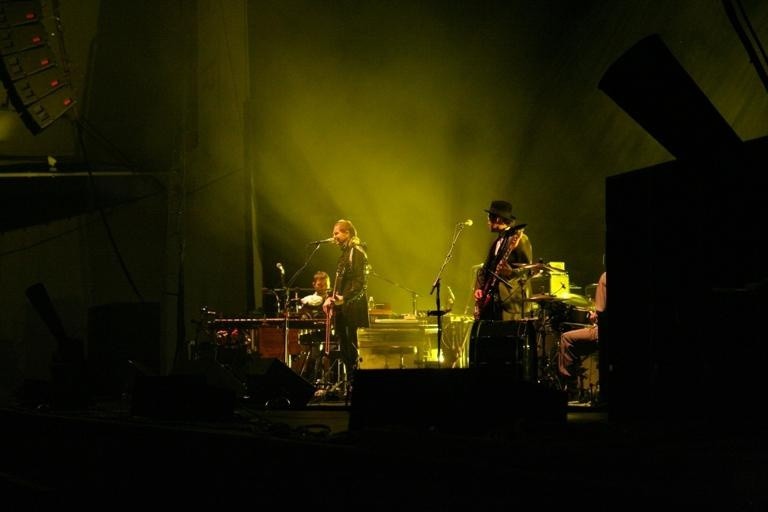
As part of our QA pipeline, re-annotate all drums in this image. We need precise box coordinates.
[549,292,595,356]
[585,283,598,302]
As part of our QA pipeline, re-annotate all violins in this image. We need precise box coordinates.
[331,252,348,299]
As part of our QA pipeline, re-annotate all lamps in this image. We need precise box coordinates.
[175,357,316,415]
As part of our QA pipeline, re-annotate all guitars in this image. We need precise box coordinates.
[473,226,526,320]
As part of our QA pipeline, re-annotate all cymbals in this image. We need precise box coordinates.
[517,293,571,301]
[569,285,582,290]
[512,262,569,275]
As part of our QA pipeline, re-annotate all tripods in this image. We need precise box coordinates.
[314,343,352,406]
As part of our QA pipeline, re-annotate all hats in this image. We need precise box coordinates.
[481,200,516,222]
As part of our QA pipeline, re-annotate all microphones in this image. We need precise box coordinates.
[275,262,285,274]
[473,262,484,268]
[458,220,473,226]
[315,238,334,243]
[447,286,455,301]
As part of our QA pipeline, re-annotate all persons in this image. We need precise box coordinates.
[559,270,609,398]
[474,199,536,319]
[324,218,367,399]
[298,271,333,384]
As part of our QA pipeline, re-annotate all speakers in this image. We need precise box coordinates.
[1,0,78,135]
[352,367,450,432]
[605,133,768,512]
[133,359,242,419]
[226,355,316,406]
[448,369,569,433]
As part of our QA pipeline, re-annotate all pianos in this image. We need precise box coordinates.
[206,317,331,330]
